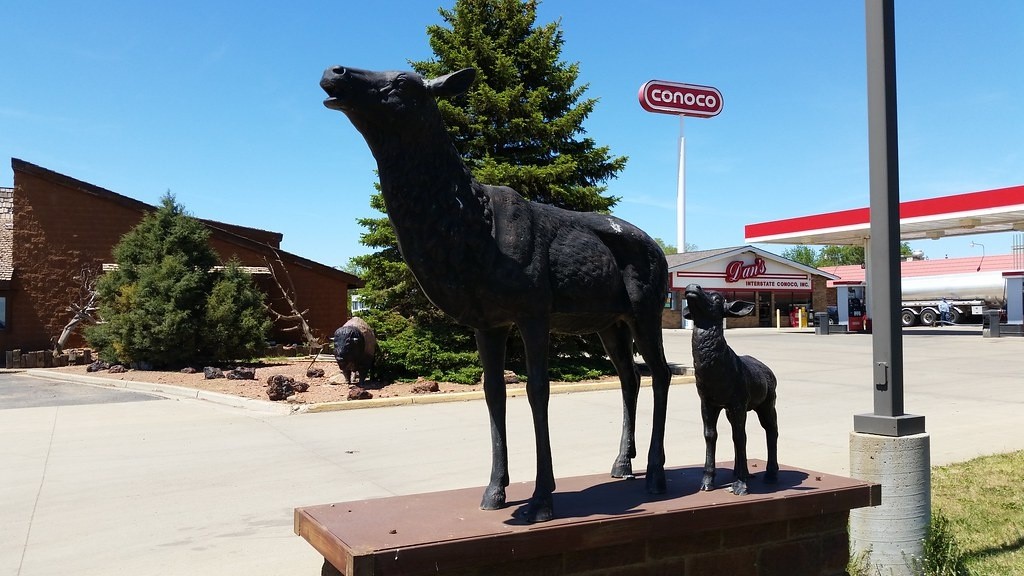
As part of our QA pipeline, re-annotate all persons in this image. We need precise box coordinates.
[938,297,950,327]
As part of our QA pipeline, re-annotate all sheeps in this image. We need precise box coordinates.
[319,63,673,523]
[330,316,375,387]
[681,283,780,495]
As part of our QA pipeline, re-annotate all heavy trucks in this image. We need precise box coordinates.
[900,269,1024,327]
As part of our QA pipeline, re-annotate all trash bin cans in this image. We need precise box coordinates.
[813,312,830,336]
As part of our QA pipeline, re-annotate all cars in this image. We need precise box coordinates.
[827,305,839,325]
